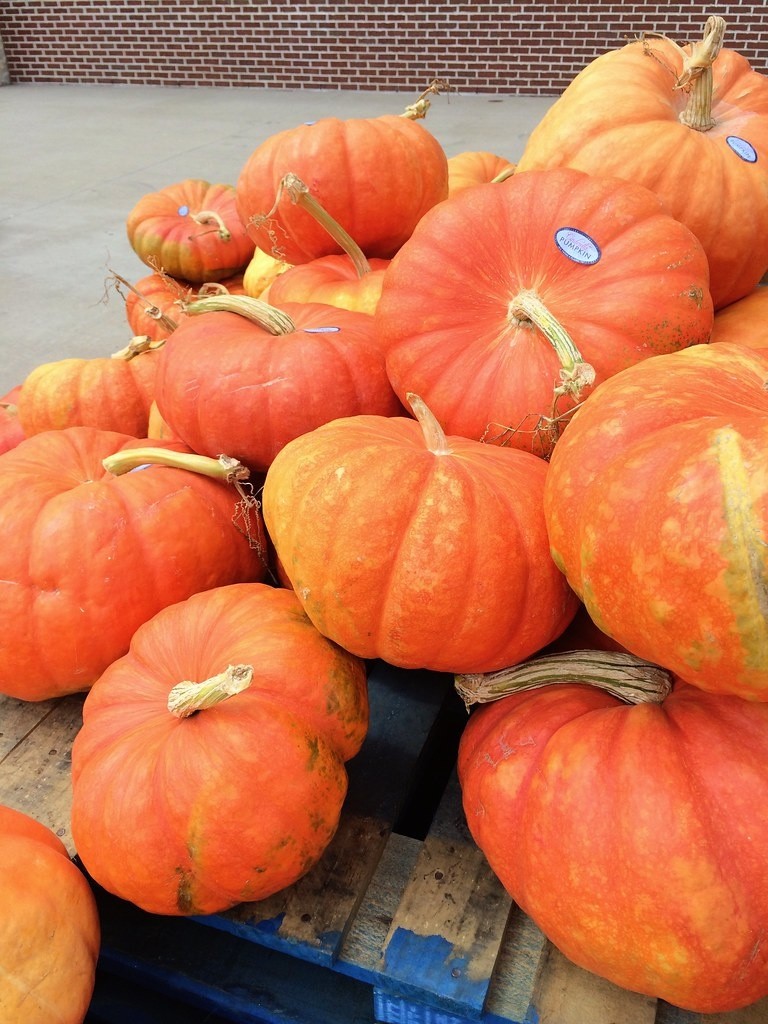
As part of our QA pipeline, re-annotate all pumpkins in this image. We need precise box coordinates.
[0,14,768,1024]
